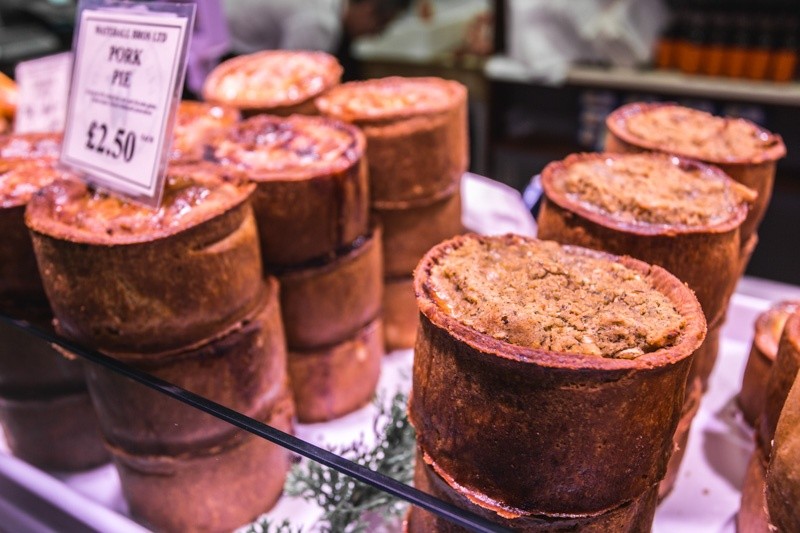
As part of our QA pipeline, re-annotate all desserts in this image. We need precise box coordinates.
[0,43,800,533]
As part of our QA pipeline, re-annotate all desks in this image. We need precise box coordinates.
[351,40,800,179]
